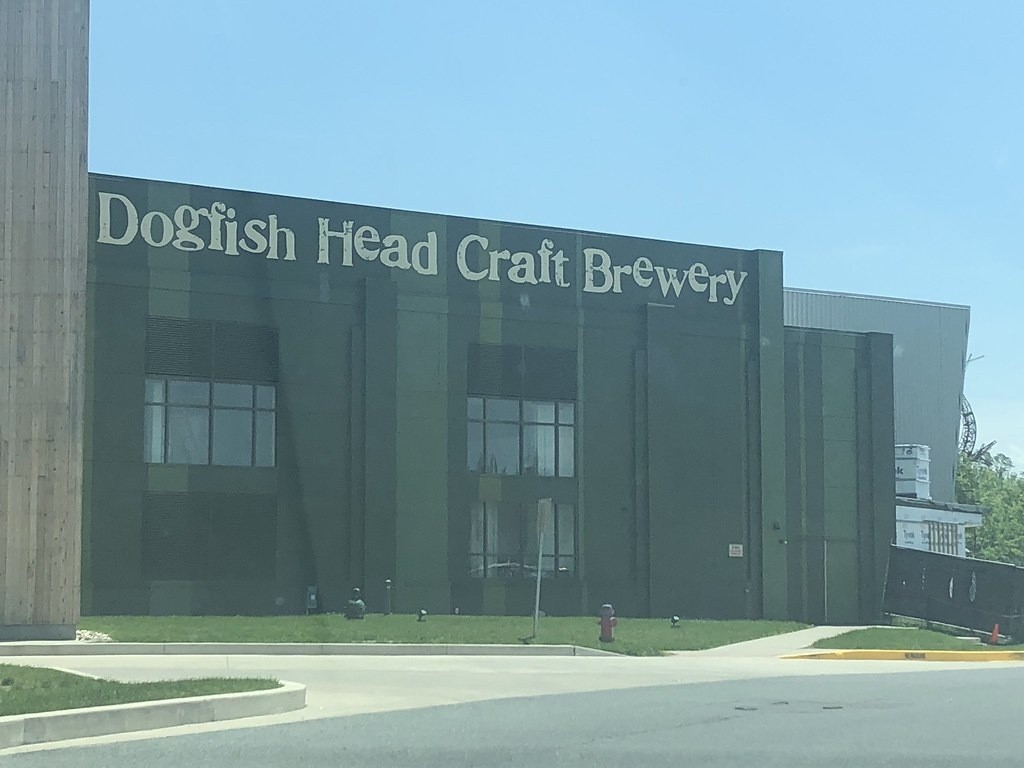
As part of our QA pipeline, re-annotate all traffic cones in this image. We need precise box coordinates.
[990,623,999,644]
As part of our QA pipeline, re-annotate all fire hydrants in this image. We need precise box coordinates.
[596,603,618,642]
